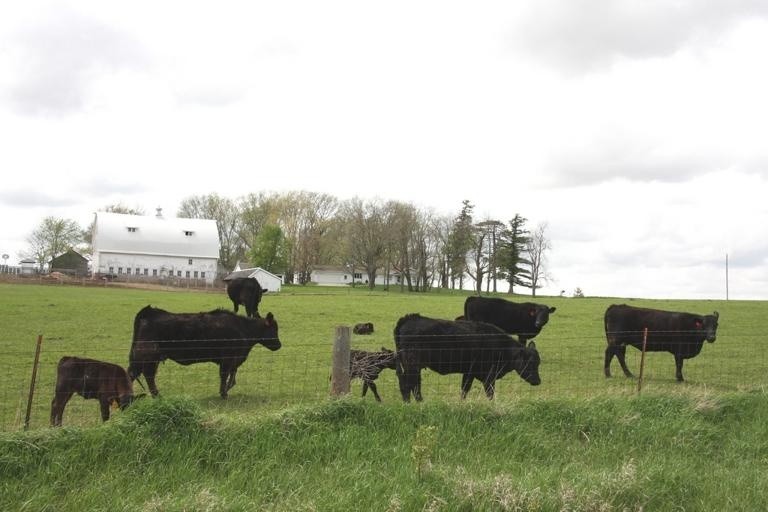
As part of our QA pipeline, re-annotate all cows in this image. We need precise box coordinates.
[127,304,282,400]
[227,277,268,319]
[329,346,397,402]
[393,313,542,404]
[464,296,557,349]
[353,322,374,335]
[49,356,147,428]
[604,304,720,382]
